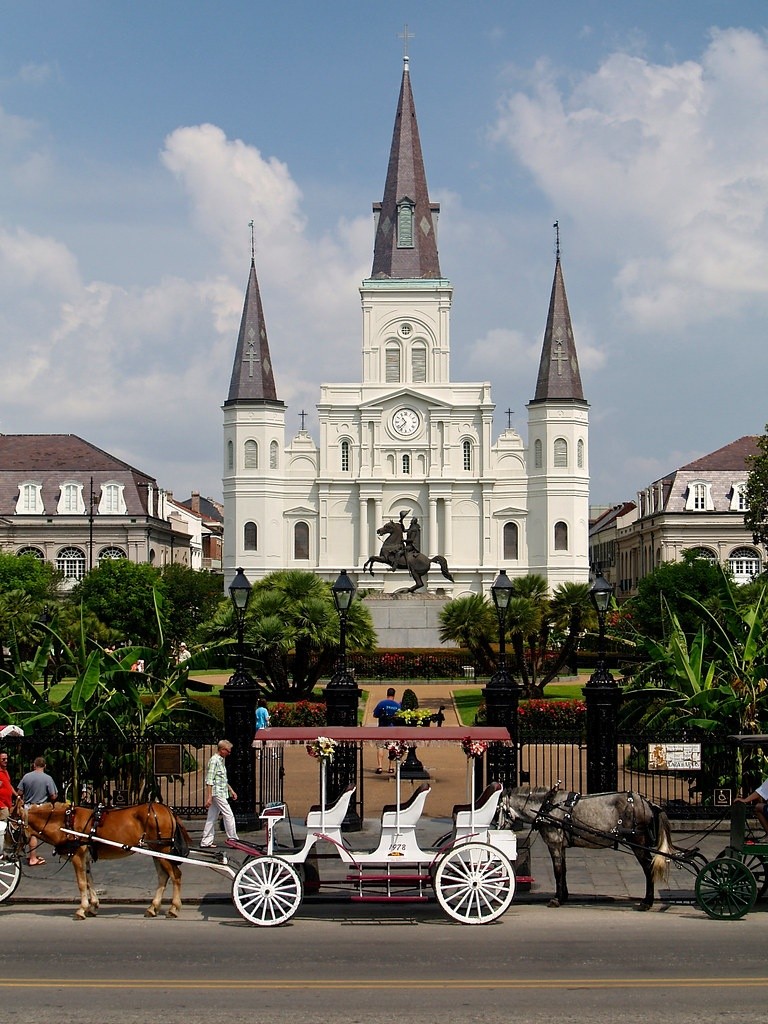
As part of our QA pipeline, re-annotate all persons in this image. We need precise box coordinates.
[176,642,191,676]
[0,753,58,867]
[734,778,768,842]
[256,699,279,759]
[131,661,141,672]
[387,517,420,572]
[373,688,402,773]
[198,740,240,848]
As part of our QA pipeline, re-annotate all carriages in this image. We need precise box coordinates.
[0,727,517,926]
[498,780,768,920]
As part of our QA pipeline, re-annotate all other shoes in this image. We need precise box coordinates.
[757,834,768,843]
[202,844,218,848]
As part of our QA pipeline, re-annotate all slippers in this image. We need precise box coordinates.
[29,858,47,866]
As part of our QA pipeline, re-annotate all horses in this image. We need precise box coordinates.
[493,785,682,913]
[364,520,455,593]
[2,795,193,921]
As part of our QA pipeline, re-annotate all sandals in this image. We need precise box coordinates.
[375,768,383,774]
[389,769,394,773]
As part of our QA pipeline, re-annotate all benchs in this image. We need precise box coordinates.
[259,801,286,818]
[381,782,432,827]
[451,782,503,826]
[305,782,357,826]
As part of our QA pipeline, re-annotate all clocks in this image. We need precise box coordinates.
[393,408,420,435]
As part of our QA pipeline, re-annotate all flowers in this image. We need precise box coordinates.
[394,707,432,727]
[461,736,488,759]
[305,737,338,764]
[382,741,410,762]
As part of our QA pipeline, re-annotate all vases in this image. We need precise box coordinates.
[392,717,430,780]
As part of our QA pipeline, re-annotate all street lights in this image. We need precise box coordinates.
[323,569,363,832]
[582,568,623,795]
[482,570,522,789]
[218,567,263,832]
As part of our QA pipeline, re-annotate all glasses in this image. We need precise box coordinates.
[2,758,8,761]
[225,748,231,754]
